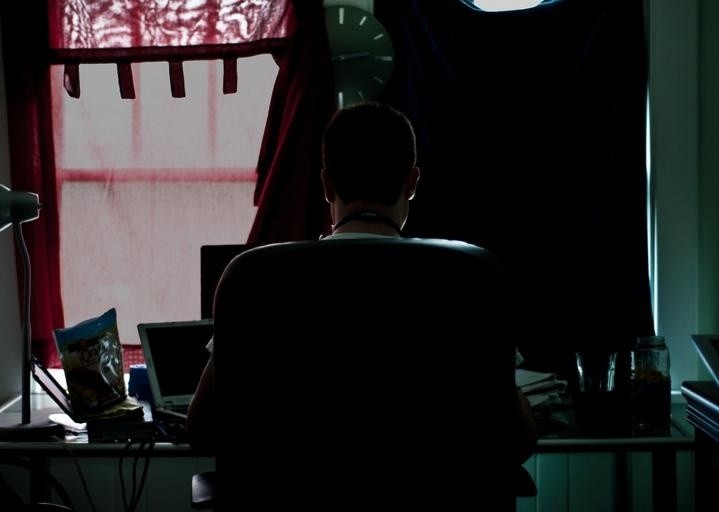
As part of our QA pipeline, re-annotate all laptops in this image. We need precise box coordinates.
[137,317,214,425]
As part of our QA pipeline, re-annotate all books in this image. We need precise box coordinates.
[515,366,568,408]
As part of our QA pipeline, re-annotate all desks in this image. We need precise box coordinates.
[0,385,697,512]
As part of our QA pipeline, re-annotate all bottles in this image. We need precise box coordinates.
[630,336,671,414]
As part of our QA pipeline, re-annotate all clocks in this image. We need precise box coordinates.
[319,4,392,114]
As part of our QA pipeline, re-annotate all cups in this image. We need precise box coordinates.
[575,351,617,393]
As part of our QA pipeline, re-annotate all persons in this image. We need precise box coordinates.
[187,105,538,464]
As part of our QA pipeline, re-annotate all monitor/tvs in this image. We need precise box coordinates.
[201,246,257,319]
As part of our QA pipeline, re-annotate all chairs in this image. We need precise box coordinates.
[210,230,521,512]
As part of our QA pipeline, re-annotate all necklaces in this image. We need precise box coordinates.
[333,209,402,235]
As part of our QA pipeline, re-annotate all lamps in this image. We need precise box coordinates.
[0,182,67,440]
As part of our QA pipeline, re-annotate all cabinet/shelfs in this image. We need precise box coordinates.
[680,381,719,512]
